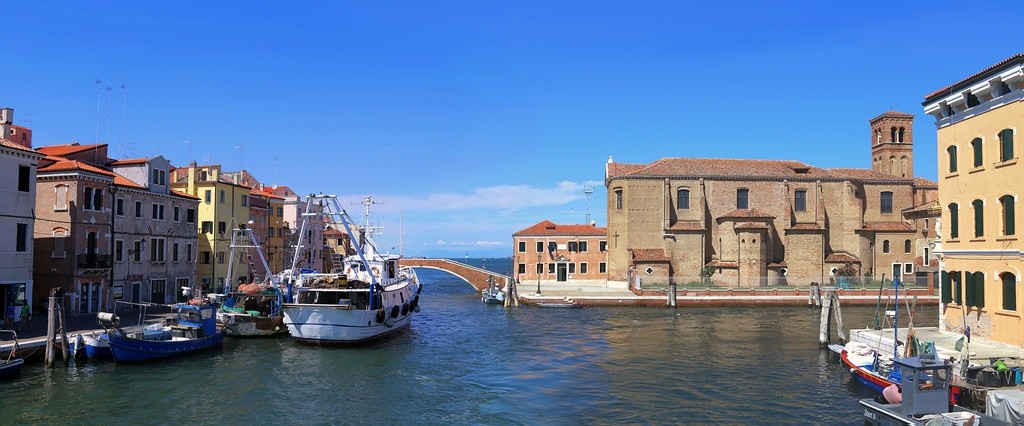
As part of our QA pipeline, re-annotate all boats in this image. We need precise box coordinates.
[481,274,505,304]
[859,351,1020,426]
[276,189,422,345]
[840,271,966,406]
[0,329,27,380]
[208,222,321,338]
[66,325,171,365]
[98,295,227,363]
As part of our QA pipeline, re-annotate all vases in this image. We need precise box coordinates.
[973,370,1017,387]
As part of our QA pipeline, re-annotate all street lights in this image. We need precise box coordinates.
[512,255,515,276]
[536,253,543,295]
[516,256,520,283]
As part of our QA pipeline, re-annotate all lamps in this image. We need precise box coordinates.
[128,237,148,256]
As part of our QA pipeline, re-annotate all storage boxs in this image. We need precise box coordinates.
[942,412,981,426]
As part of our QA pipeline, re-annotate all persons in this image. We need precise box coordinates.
[17,299,31,332]
[7,301,15,332]
